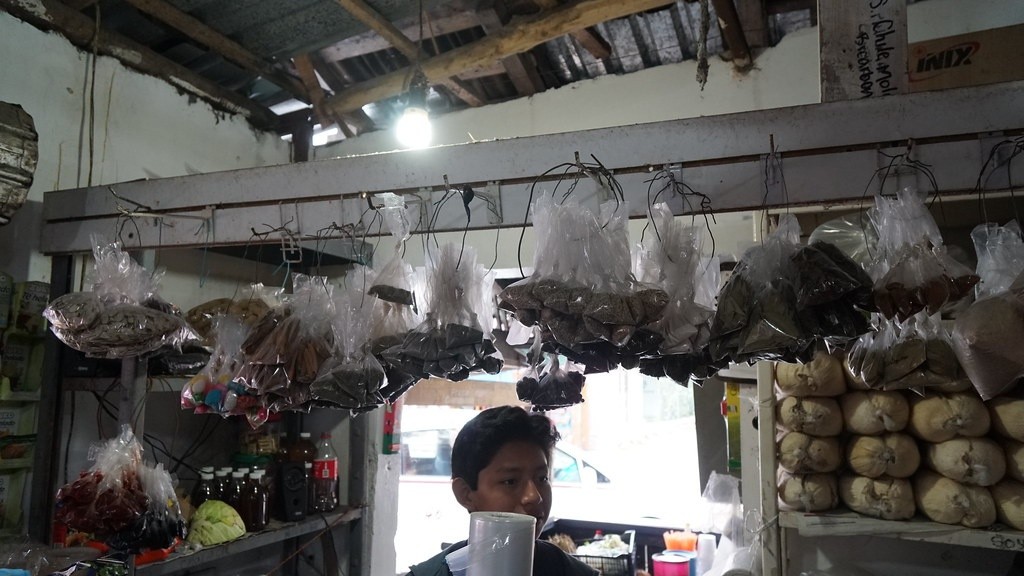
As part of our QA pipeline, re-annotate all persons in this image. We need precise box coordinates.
[402,406,600,576]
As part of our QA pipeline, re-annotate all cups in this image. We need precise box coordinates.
[652,533,716,576]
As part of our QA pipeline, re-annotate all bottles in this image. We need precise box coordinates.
[594,529,602,541]
[192,413,338,533]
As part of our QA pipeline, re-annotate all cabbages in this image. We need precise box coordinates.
[186,500,246,546]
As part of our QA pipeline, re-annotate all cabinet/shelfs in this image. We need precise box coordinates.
[29,234,376,576]
[753,191,1023,576]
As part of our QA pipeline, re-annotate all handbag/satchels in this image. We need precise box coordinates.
[42,185,1023,429]
[54,424,189,556]
